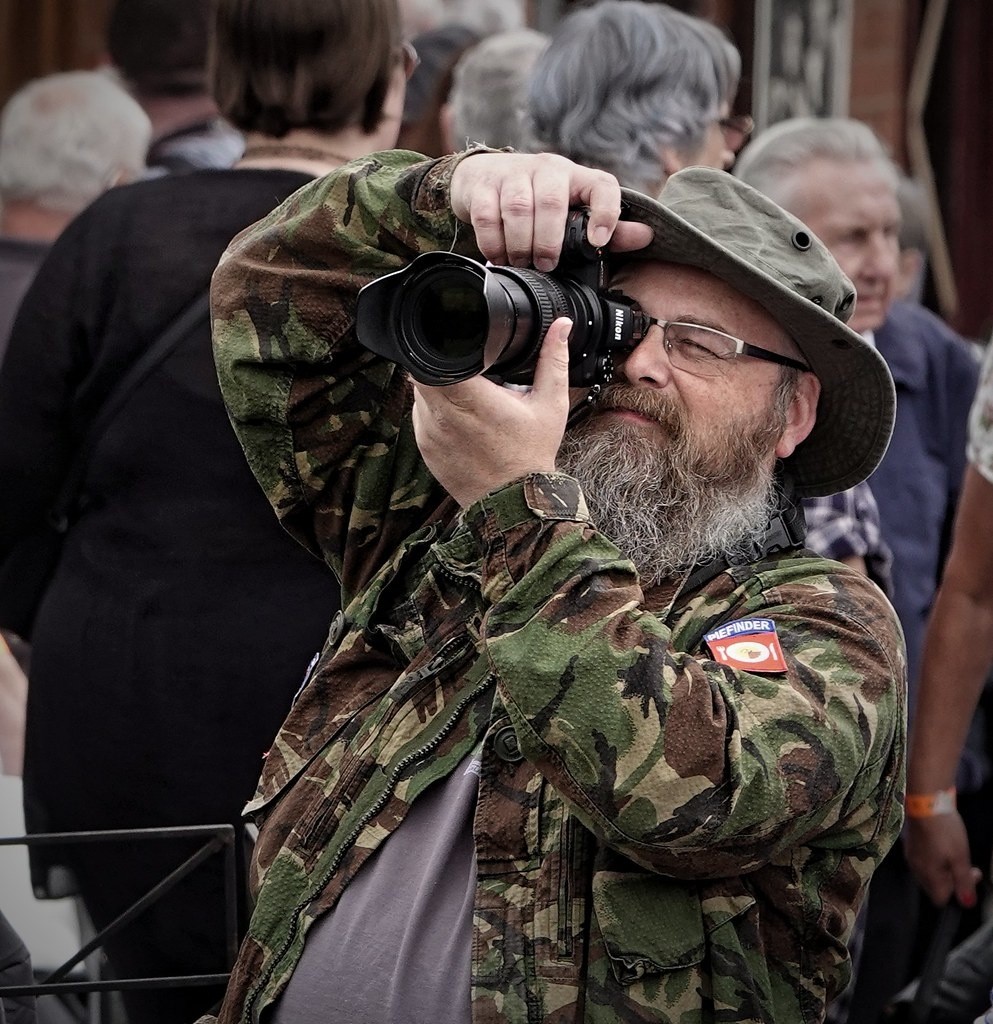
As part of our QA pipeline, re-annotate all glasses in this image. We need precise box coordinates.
[394,40,421,79]
[716,111,756,137]
[641,314,812,376]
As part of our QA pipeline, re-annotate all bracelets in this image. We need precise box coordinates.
[905,784,959,818]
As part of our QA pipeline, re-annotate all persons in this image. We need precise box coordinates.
[0,0,993,1024]
[208,143,909,1024]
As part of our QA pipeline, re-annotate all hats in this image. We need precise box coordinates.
[621,164,899,500]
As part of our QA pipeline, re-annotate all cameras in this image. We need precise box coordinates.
[355,201,649,386]
